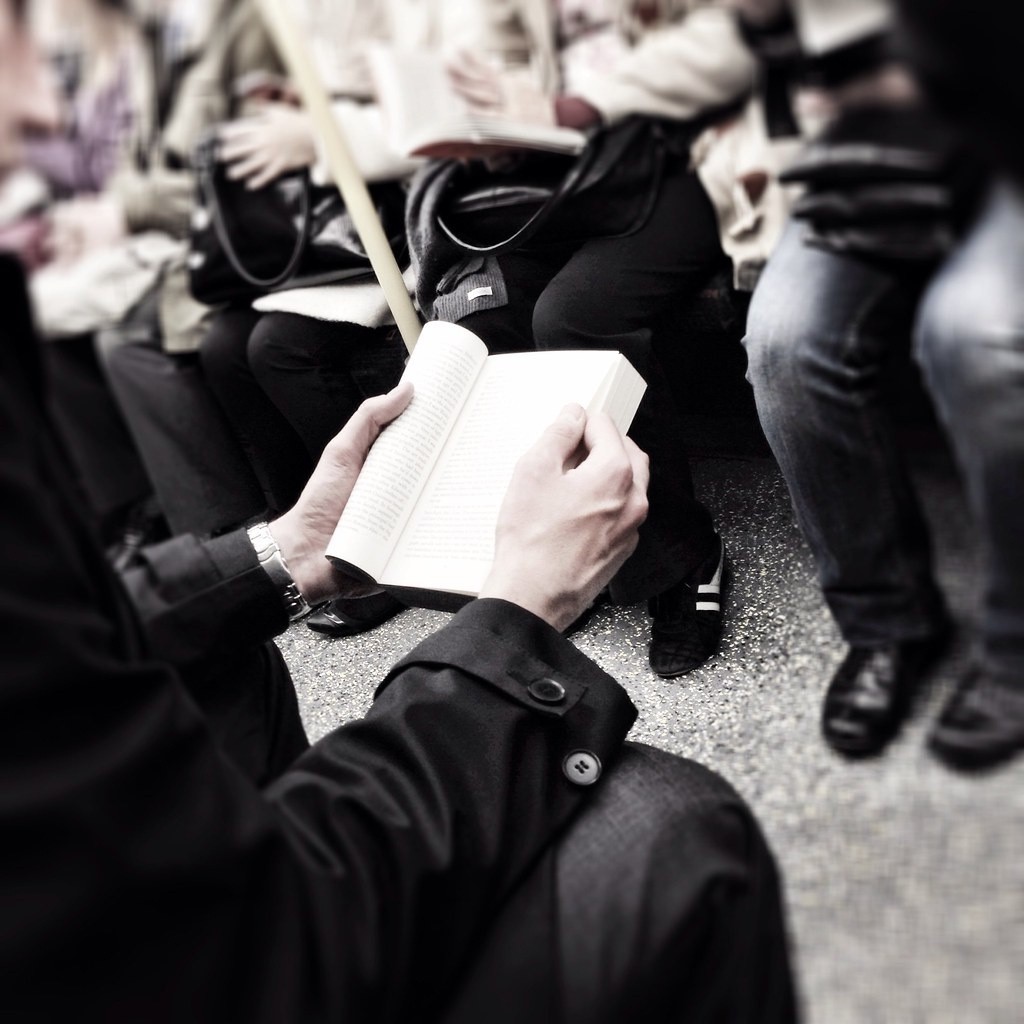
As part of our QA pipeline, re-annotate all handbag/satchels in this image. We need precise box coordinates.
[431,111,667,254]
[189,132,410,304]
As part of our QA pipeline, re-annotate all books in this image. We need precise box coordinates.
[316,314,654,603]
[409,116,587,166]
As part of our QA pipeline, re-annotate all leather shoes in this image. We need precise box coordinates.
[307,600,402,635]
[931,667,1023,769]
[826,620,955,760]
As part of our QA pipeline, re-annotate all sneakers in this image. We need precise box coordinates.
[651,532,727,676]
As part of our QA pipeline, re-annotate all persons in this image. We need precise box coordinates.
[3,7,293,600]
[296,0,804,681]
[3,6,159,512]
[0,2,804,1020]
[729,0,1024,775]
